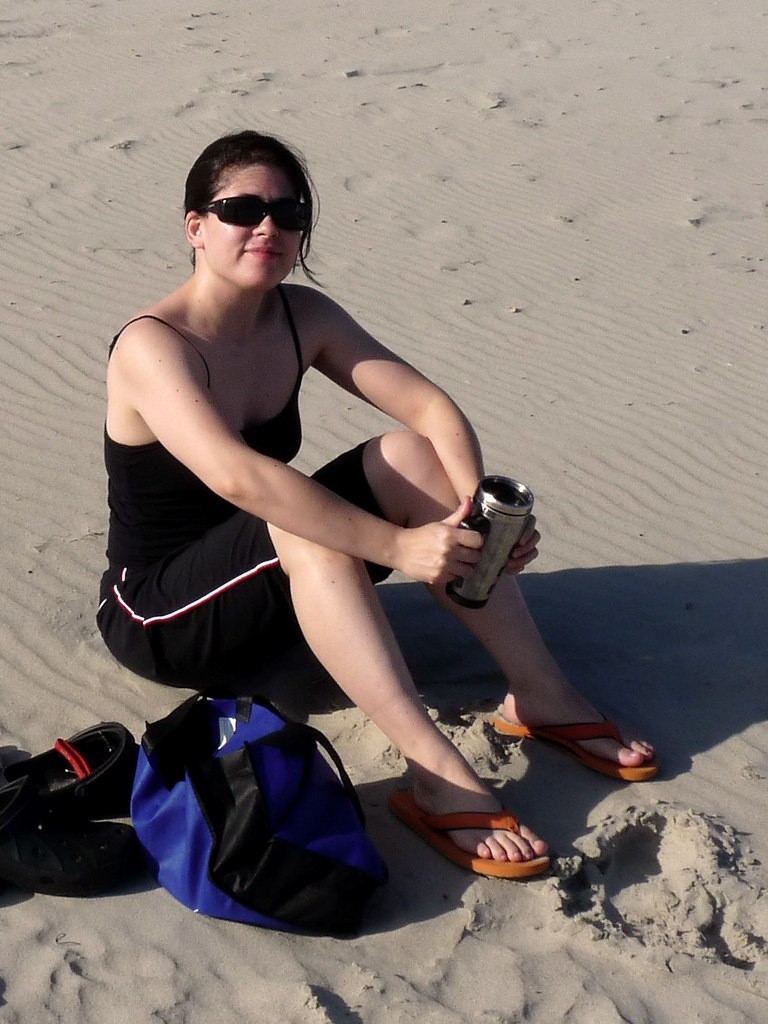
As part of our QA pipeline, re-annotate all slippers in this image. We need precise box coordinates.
[493,708,659,782]
[388,791,550,878]
[0,722,140,818]
[0,775,141,898]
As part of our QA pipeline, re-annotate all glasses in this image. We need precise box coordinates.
[197,195,311,231]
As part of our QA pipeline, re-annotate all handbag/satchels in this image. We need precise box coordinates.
[131,688,389,940]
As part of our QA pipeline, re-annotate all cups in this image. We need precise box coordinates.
[444,475,534,611]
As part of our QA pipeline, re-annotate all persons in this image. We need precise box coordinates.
[92,132,658,877]
